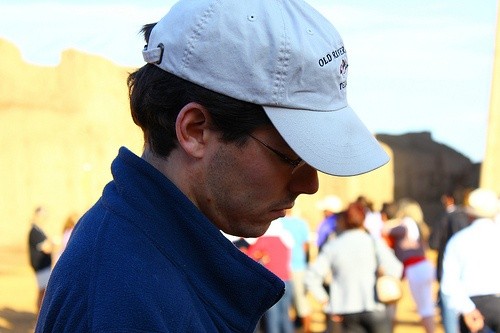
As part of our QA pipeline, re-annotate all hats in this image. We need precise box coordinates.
[468,187,499,216]
[142,0,391,177]
[317,196,343,214]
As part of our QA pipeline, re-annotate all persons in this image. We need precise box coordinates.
[34,0,390,333]
[27,187,500,333]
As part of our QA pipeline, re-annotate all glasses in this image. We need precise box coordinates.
[237,125,310,175]
